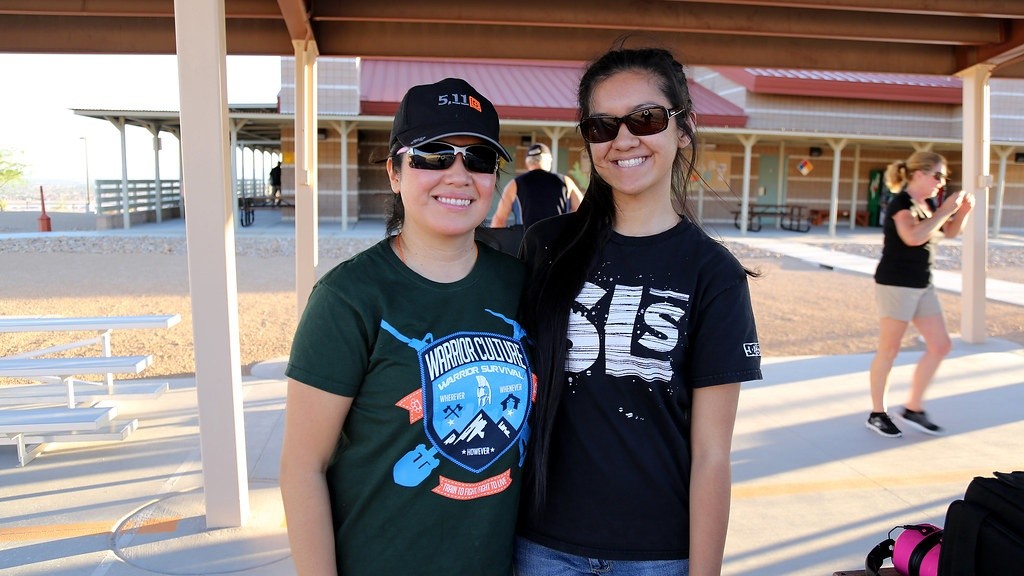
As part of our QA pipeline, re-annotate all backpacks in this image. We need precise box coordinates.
[864,471,1024,576]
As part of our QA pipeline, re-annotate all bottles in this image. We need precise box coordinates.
[891,522,944,576]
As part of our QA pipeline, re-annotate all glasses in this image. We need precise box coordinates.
[396,142,499,174]
[925,172,948,181]
[579,105,685,143]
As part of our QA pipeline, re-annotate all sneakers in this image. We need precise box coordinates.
[866,411,902,437]
[900,408,943,435]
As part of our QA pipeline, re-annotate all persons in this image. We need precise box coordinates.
[866,155,978,438]
[518,47,762,576]
[270,162,282,209]
[490,144,583,229]
[278,78,536,576]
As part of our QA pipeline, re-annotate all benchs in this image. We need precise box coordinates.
[730,211,811,232]
[810,209,869,227]
[0,419,139,445]
[0,383,169,405]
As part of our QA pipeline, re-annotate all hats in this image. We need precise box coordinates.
[527,142,552,156]
[388,78,512,163]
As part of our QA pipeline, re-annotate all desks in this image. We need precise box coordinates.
[239,195,295,227]
[0,314,182,385]
[738,204,807,232]
[0,407,117,433]
[240,204,295,227]
[0,355,153,409]
